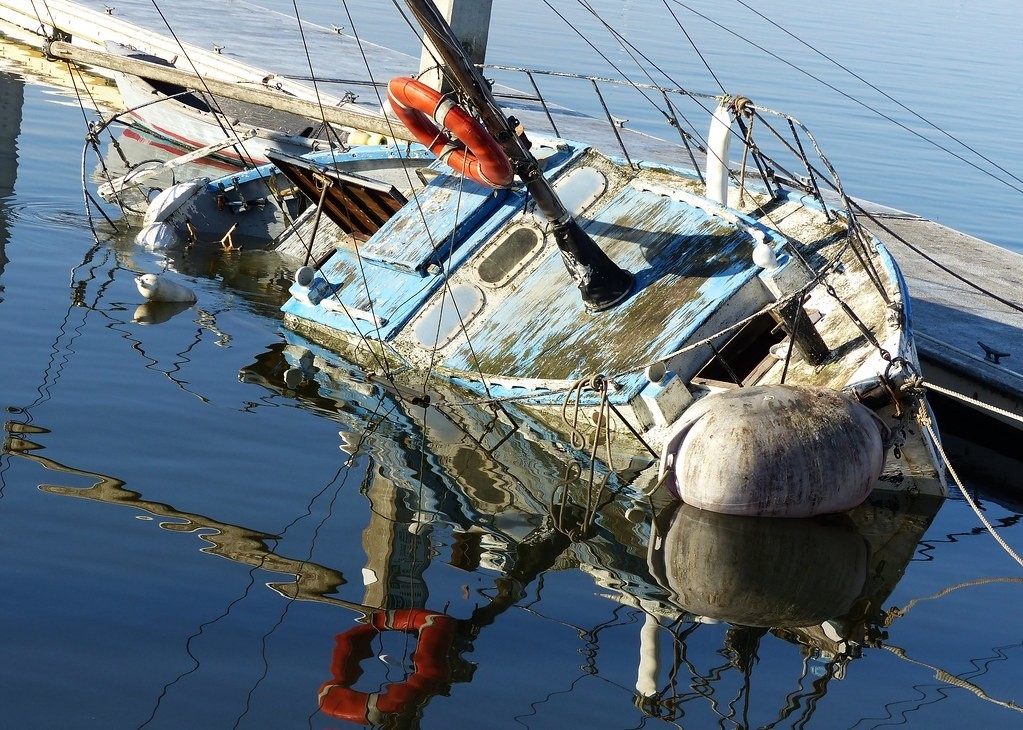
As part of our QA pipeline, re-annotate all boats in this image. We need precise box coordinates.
[100,37,374,177]
[0,0,949,520]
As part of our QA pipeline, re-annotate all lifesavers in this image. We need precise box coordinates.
[385,75,516,192]
[317,608,462,722]
